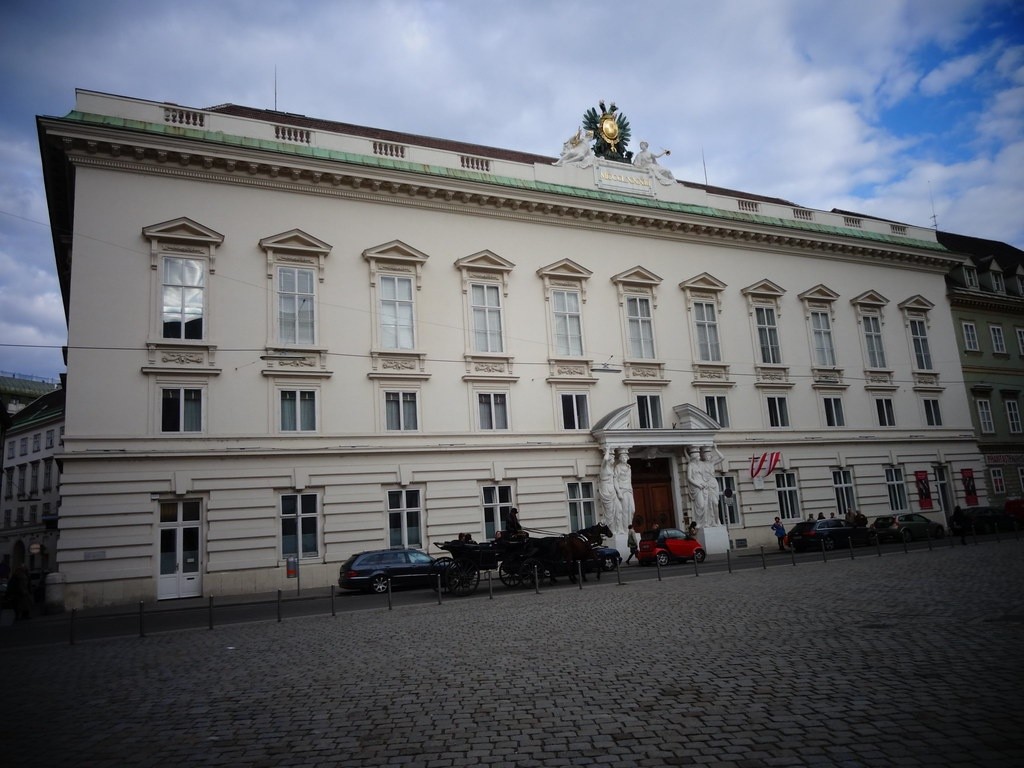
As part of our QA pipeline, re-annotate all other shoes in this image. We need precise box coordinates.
[626,561,630,565]
[961,539,967,545]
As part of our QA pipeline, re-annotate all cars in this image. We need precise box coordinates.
[636,528,706,568]
[949,506,1016,538]
[786,517,870,553]
[868,512,959,541]
[516,544,623,582]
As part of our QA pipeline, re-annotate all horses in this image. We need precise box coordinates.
[527,522,614,588]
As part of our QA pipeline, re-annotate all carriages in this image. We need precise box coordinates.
[426,522,613,594]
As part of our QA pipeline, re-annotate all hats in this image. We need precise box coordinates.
[511,508,518,513]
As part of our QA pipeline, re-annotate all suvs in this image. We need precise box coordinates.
[338,546,475,594]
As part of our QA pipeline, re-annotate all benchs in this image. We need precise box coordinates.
[446,540,482,565]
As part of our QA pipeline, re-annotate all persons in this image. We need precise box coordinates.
[598,453,635,533]
[495,531,502,539]
[683,444,725,528]
[625,524,641,564]
[689,521,698,539]
[458,533,478,544]
[551,125,594,165]
[807,508,868,527]
[633,141,676,186]
[771,517,787,550]
[506,508,521,533]
[6,563,34,619]
[951,505,967,545]
